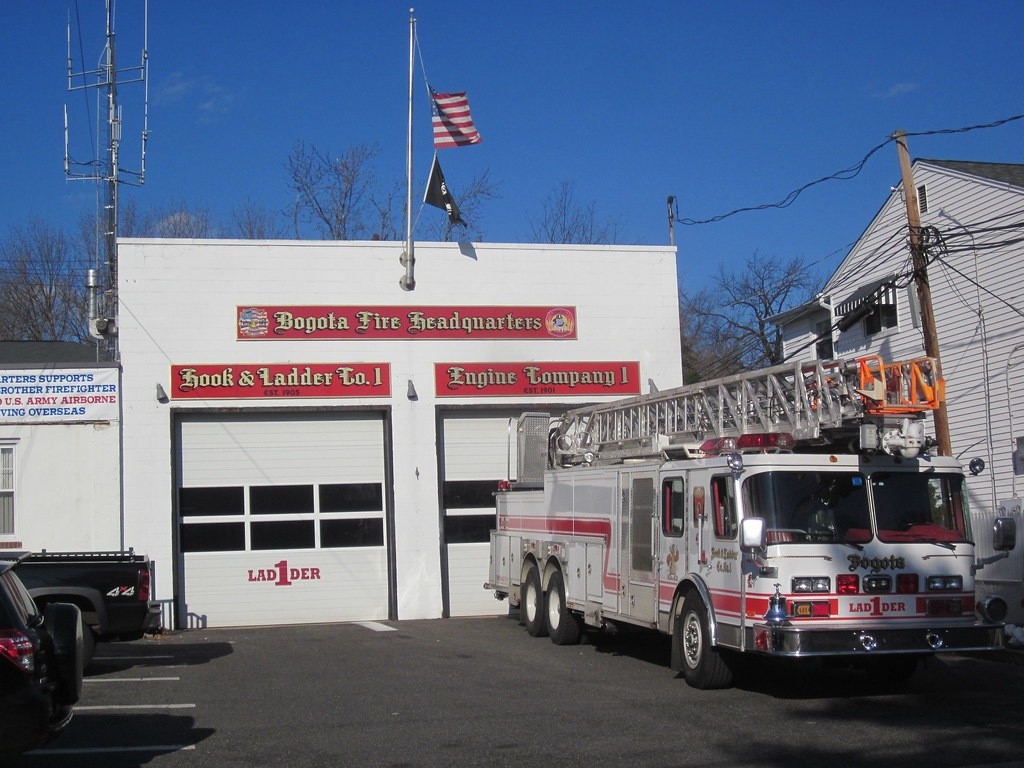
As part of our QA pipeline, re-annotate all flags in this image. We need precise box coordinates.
[425,156,468,229]
[429,86,483,149]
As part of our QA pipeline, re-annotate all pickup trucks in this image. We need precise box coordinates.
[0,549,163,665]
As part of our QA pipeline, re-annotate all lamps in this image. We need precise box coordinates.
[407,379,416,398]
[648,377,659,395]
[156,383,167,400]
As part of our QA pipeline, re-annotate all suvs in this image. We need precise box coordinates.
[0,561,84,758]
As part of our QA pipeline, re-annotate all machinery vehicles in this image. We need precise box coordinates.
[483,355,1016,690]
